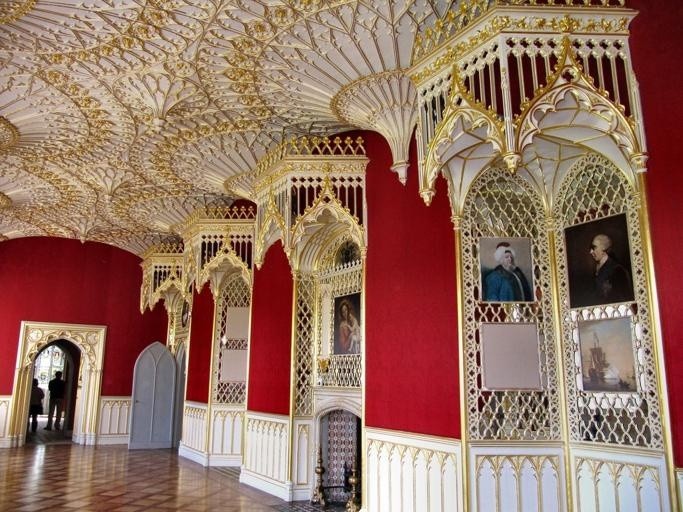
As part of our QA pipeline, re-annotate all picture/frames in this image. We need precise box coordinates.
[562,212,638,310]
[577,315,640,394]
[479,322,544,392]
[333,292,361,355]
[477,237,536,304]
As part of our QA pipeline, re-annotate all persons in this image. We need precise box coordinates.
[590,234,633,303]
[485,242,532,301]
[43,371,64,432]
[27,379,45,433]
[338,300,360,354]
